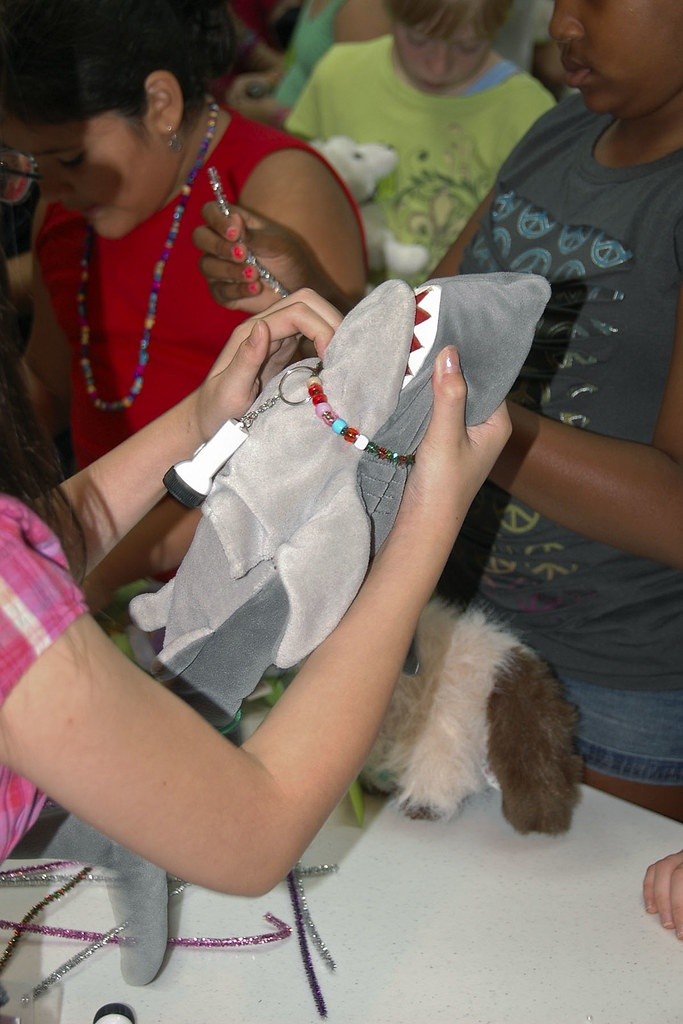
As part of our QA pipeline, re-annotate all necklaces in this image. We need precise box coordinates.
[309,360,423,471]
[77,96,218,410]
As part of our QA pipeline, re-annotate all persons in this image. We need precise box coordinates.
[0,0,683,932]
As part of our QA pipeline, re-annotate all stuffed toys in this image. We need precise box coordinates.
[298,132,427,293]
[10,272,584,986]
[356,594,587,837]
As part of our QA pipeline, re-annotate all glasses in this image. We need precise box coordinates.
[0,149,43,205]
